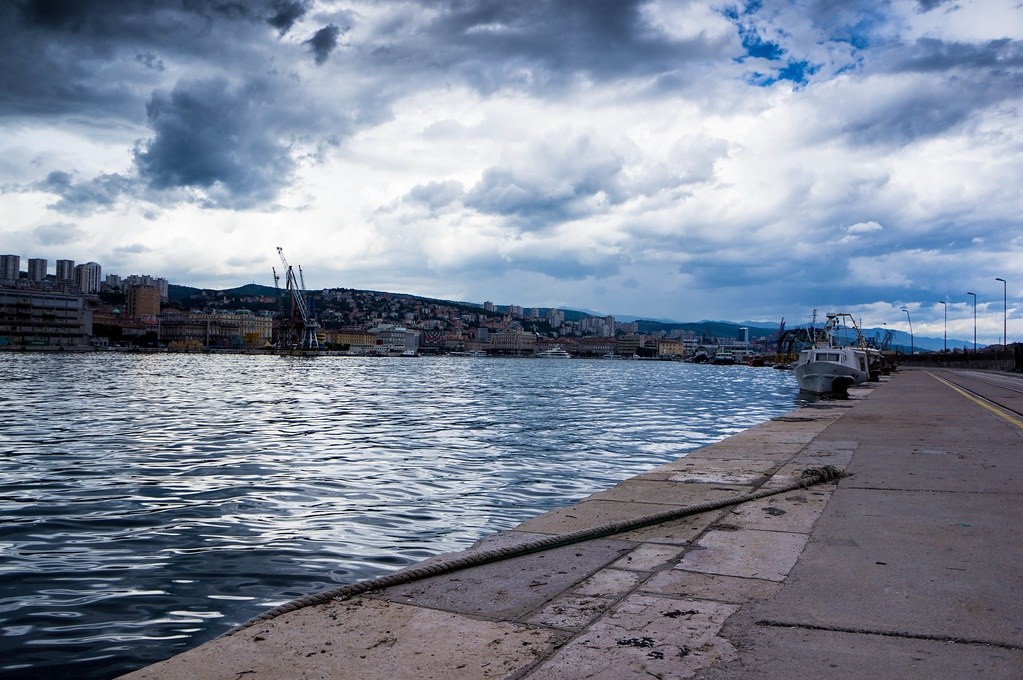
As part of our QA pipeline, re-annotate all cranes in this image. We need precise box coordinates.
[272,247,320,348]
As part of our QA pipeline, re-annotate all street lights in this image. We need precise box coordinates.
[902,309,914,355]
[995,278,1007,361]
[967,292,977,356]
[940,300,947,354]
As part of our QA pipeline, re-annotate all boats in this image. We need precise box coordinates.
[795,312,869,392]
[536,347,573,360]
[400,349,420,357]
